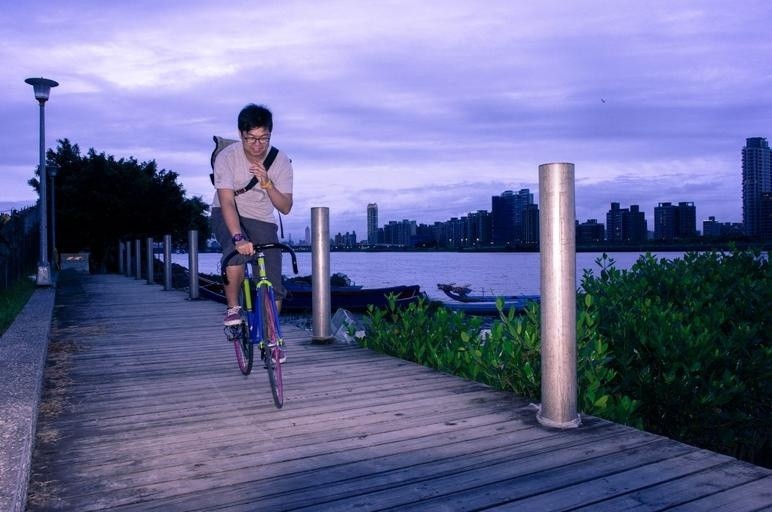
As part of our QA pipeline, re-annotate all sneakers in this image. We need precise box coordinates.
[271,345,287,364]
[223,304,243,327]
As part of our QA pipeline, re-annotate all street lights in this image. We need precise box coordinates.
[22,74,59,291]
[45,161,60,271]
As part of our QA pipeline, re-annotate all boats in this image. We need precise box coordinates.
[436,281,541,315]
[186,259,435,315]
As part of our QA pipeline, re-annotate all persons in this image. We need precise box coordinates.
[212,101,293,365]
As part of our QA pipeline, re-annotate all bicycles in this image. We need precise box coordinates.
[215,238,304,411]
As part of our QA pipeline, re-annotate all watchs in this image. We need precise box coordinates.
[261,178,273,189]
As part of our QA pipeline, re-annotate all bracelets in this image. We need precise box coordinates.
[232,231,246,244]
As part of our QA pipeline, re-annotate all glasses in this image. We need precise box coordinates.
[243,134,270,144]
